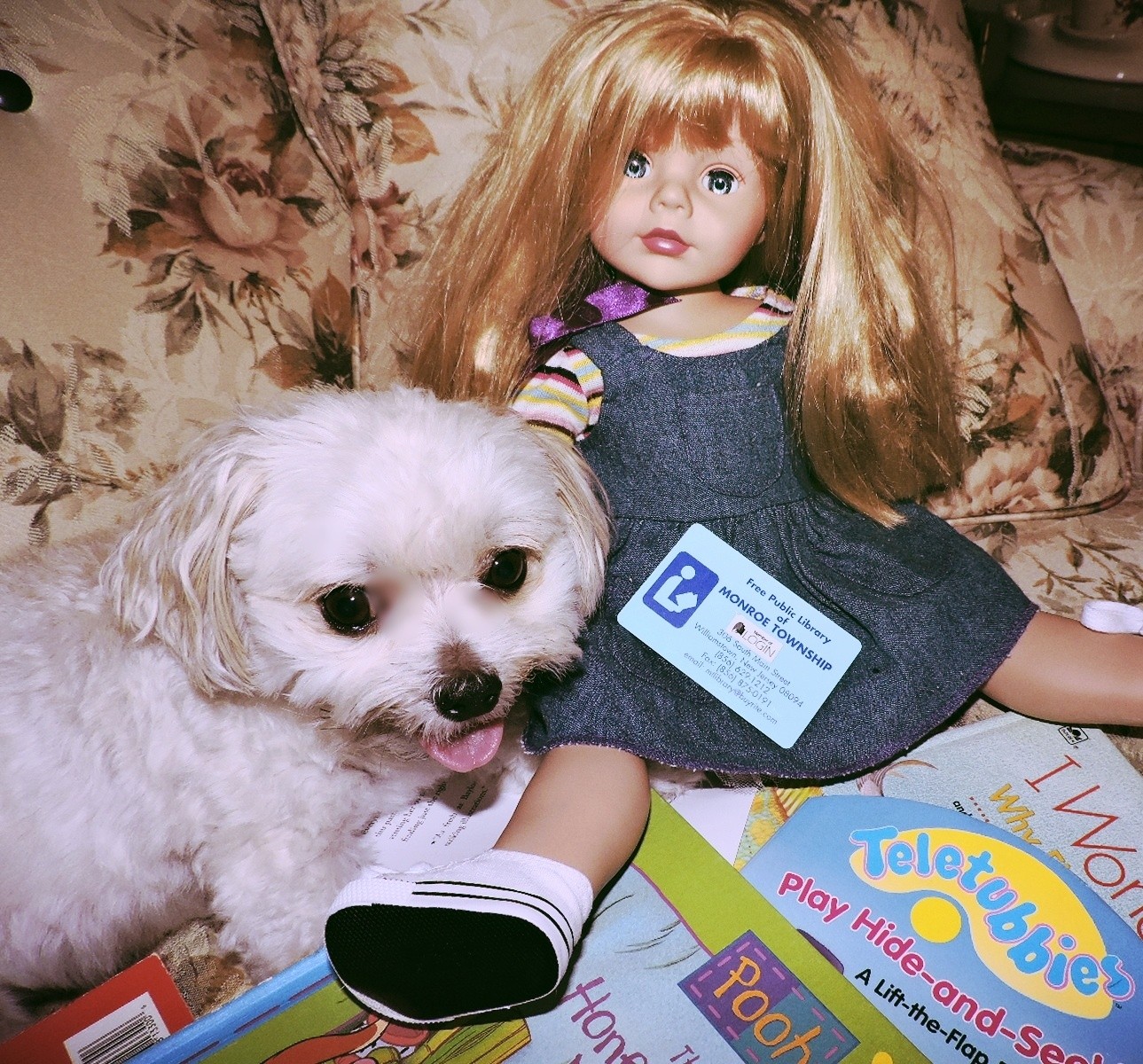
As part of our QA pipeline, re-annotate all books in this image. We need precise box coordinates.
[120,786,937,1064]
[733,714,1143,941]
[0,952,196,1064]
[743,793,1143,1064]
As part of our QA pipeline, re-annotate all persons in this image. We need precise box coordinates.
[324,0,1143,1024]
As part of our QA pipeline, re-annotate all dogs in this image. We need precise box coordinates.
[3,384,618,987]
